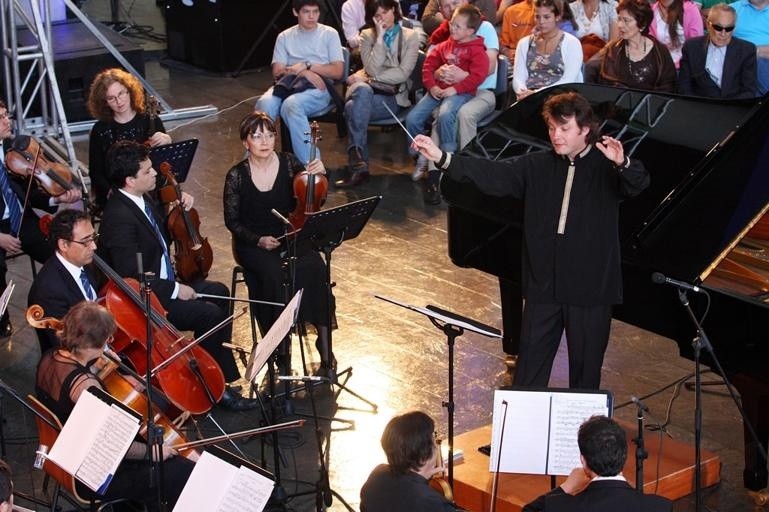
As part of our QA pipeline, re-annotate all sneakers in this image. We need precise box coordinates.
[412,163,427,181]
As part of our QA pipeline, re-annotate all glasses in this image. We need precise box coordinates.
[106,92,129,103]
[73,234,100,246]
[249,133,274,141]
[712,25,734,32]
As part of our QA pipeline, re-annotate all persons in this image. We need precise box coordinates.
[88,68,173,220]
[34,300,195,502]
[520,414,673,512]
[359,411,468,512]
[96,139,260,412]
[1,102,84,341]
[223,110,338,373]
[27,208,110,355]
[409,92,651,393]
[0,459,14,512]
[254,0,345,172]
[335,0,769,205]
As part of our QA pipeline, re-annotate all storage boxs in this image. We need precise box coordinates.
[0,14,147,125]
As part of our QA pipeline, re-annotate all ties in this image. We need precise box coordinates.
[0,159,21,233]
[80,270,93,300]
[145,202,175,282]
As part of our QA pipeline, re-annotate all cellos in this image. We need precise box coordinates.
[25,304,193,458]
[40,214,225,418]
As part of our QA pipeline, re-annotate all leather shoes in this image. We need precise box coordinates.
[217,386,256,412]
[423,183,442,205]
[0,322,12,337]
[335,171,369,187]
[316,338,336,369]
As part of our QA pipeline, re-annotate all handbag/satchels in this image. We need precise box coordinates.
[273,74,311,99]
[369,79,399,95]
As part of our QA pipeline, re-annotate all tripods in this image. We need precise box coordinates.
[240,256,355,444]
[268,248,379,411]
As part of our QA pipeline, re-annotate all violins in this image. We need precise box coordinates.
[6,137,98,213]
[160,162,213,283]
[286,121,328,239]
[429,432,454,501]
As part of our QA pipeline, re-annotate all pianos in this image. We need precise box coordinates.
[439,83,766,494]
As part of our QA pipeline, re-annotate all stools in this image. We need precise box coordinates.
[229,267,258,346]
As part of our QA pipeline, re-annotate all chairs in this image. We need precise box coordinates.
[25,394,150,512]
[5,246,38,284]
[368,50,426,126]
[279,46,349,156]
[477,54,509,128]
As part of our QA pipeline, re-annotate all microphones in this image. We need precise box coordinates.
[652,273,703,294]
[630,394,650,412]
